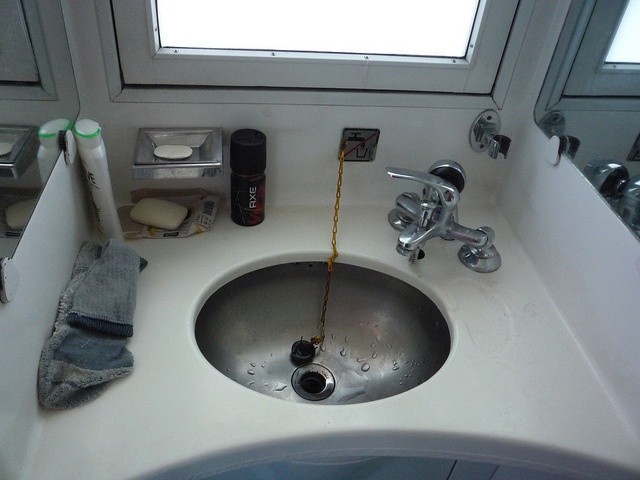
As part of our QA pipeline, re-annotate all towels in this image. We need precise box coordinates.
[36,235,150,412]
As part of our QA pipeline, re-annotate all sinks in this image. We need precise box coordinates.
[189,248,455,405]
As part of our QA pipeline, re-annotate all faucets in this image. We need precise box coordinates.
[615,180,640,233]
[384,158,502,273]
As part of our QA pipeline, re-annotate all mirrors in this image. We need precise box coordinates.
[0,0,83,261]
[532,0,636,243]
